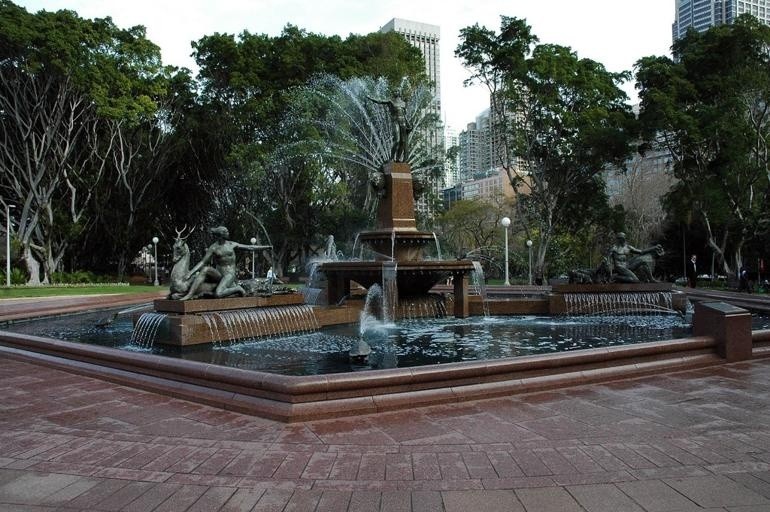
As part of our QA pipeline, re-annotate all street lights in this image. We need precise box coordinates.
[502,217,512,285]
[6,205,16,287]
[250,238,257,278]
[139,236,159,286]
[526,240,532,283]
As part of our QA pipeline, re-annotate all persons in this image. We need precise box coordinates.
[178,225,273,302]
[364,87,407,162]
[266,266,284,284]
[446,275,454,286]
[599,232,664,284]
[396,122,413,163]
[737,258,753,294]
[686,254,698,288]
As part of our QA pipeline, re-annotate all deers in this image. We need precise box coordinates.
[167,223,218,299]
